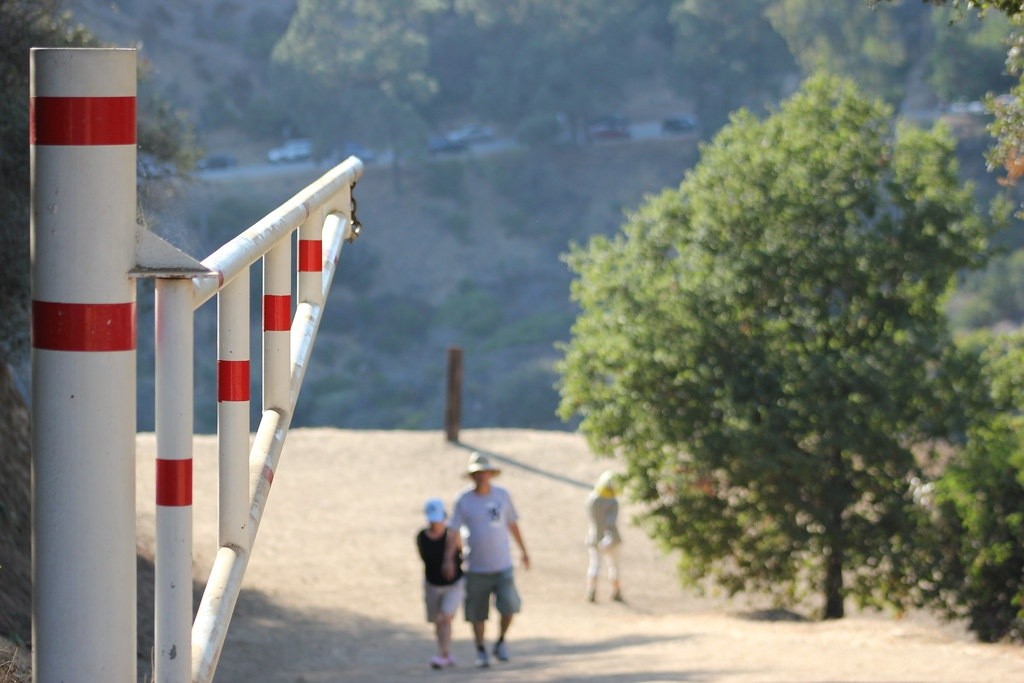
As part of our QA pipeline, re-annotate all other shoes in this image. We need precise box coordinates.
[493,642,508,661]
[613,593,623,602]
[589,594,595,602]
[429,654,458,669]
[474,647,488,667]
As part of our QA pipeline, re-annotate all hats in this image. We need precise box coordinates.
[595,484,614,499]
[425,500,447,522]
[461,453,501,476]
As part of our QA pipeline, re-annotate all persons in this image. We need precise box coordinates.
[440,450,533,669]
[416,497,465,667]
[583,485,622,604]
